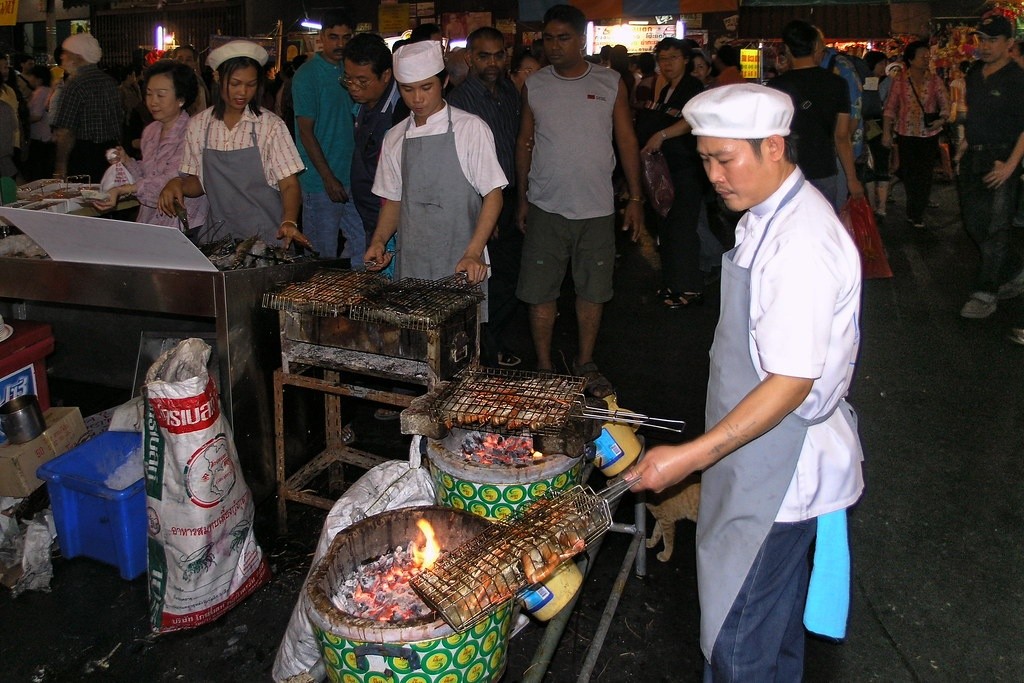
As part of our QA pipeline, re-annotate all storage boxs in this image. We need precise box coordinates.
[37,431,165,581]
[0,407,91,497]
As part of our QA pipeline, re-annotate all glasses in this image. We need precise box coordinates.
[514,69,533,76]
[915,55,932,61]
[337,70,382,90]
[657,53,682,64]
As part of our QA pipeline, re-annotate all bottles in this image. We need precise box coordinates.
[0,314,4,337]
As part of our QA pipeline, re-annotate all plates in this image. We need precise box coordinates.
[80,190,107,202]
[0,324,13,342]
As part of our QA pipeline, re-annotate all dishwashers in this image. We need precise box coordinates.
[0,395,46,443]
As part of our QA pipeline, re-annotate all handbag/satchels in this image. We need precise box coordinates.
[837,183,893,279]
[100,148,134,200]
[924,112,940,127]
[642,150,675,218]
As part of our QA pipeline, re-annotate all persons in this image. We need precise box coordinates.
[619,83,863,680]
[0,5,1024,398]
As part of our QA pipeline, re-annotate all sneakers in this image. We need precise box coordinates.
[996,268,1024,300]
[960,290,998,318]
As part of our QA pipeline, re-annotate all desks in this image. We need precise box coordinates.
[0,316,55,411]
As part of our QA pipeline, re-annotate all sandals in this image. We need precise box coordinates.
[536,361,557,380]
[573,359,612,398]
[655,276,707,307]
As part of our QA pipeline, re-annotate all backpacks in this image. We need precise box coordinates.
[826,51,882,120]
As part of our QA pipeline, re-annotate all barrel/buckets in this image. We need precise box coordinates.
[592,393,642,476]
[513,542,583,620]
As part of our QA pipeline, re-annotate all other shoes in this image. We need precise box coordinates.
[906,215,926,228]
[496,352,521,367]
[374,408,400,420]
[1008,327,1024,345]
[339,424,355,445]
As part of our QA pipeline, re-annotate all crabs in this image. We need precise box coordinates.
[439,375,576,430]
[281,271,379,312]
[419,498,589,627]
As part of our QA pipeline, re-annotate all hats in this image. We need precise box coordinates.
[885,62,904,77]
[61,31,102,64]
[392,40,446,84]
[204,40,269,71]
[968,15,1013,39]
[681,83,794,139]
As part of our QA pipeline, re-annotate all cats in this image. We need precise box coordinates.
[646,483,701,562]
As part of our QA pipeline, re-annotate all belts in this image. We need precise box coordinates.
[967,143,1012,151]
